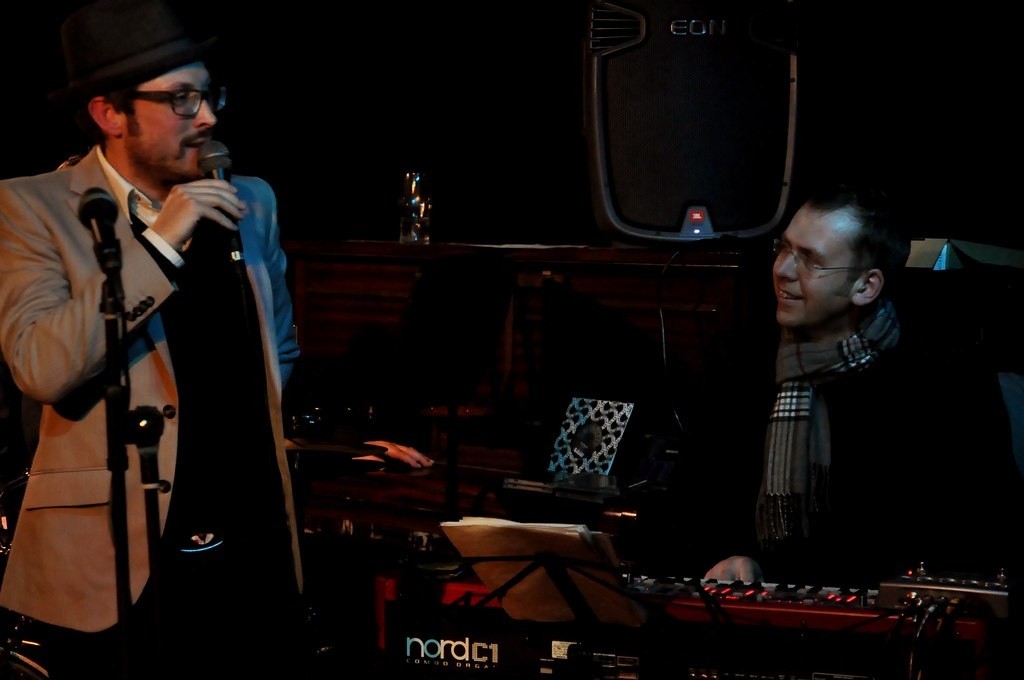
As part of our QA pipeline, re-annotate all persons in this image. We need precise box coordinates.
[0,0,306,680]
[610,194,1015,586]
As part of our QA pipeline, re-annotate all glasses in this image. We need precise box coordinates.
[129,85,227,116]
[773,238,856,279]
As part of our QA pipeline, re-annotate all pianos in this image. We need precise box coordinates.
[375,550,1009,679]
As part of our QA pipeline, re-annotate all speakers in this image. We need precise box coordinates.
[582,0,798,248]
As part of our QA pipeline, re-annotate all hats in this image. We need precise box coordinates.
[47,0,218,103]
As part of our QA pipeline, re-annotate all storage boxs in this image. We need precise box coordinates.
[905,239,1024,271]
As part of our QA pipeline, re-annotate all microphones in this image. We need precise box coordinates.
[194,141,244,265]
[78,186,124,302]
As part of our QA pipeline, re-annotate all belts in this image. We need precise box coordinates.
[176,528,225,556]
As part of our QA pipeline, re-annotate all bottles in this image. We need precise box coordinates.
[399,172,433,246]
